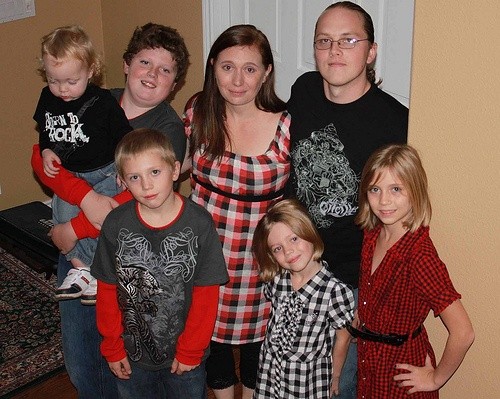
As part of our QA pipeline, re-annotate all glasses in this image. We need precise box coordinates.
[314,38,368,50]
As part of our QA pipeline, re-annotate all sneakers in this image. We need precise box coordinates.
[54,269,94,299]
[81,276,97,305]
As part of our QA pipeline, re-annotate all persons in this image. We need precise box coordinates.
[284,1,409,399]
[350,143,475,399]
[32,24,136,305]
[252,198,356,399]
[30,20,191,399]
[179,24,290,399]
[89,128,231,399]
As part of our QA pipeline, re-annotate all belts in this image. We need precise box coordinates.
[346,321,422,347]
